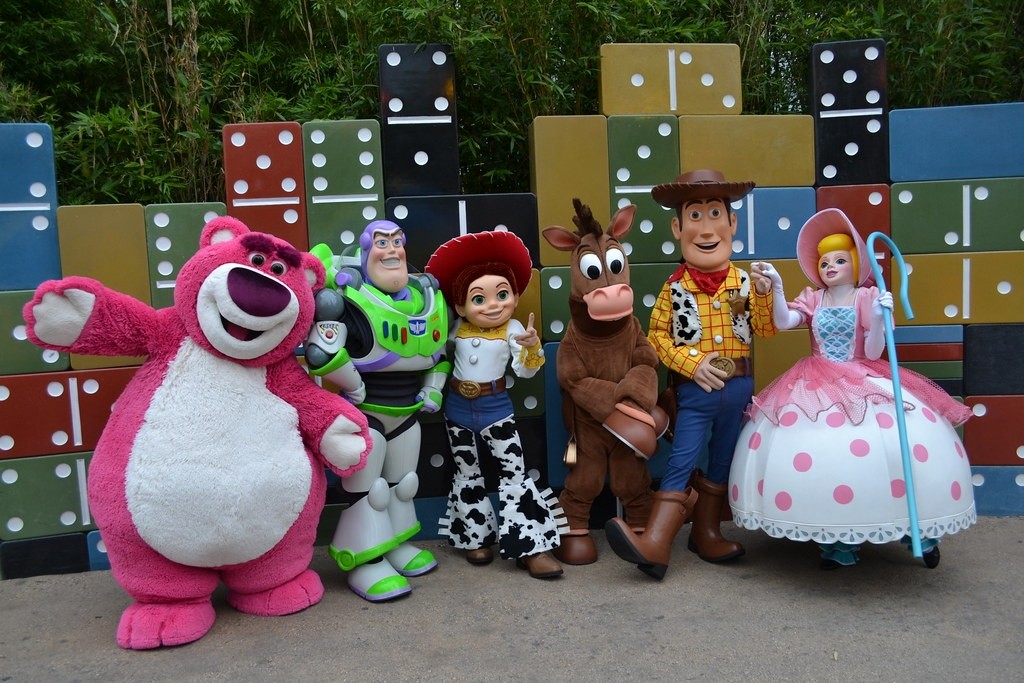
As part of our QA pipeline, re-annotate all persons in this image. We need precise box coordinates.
[729,208,977,569]
[306,220,450,602]
[605,170,780,580]
[424,231,563,578]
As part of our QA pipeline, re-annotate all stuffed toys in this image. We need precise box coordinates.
[542,199,669,565]
[24,216,371,651]
